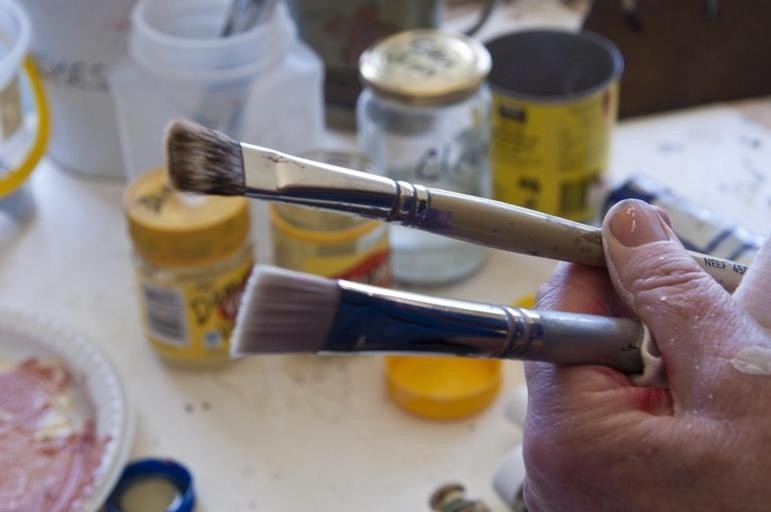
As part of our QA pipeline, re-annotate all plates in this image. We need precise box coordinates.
[0,298,132,512]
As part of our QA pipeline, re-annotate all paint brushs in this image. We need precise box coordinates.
[232,262,638,375]
[167,119,750,295]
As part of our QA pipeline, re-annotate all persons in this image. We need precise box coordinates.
[521,197,771,512]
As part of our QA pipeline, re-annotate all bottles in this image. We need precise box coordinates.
[22,4,132,184]
[0,0,47,249]
[354,29,492,287]
[106,1,332,178]
